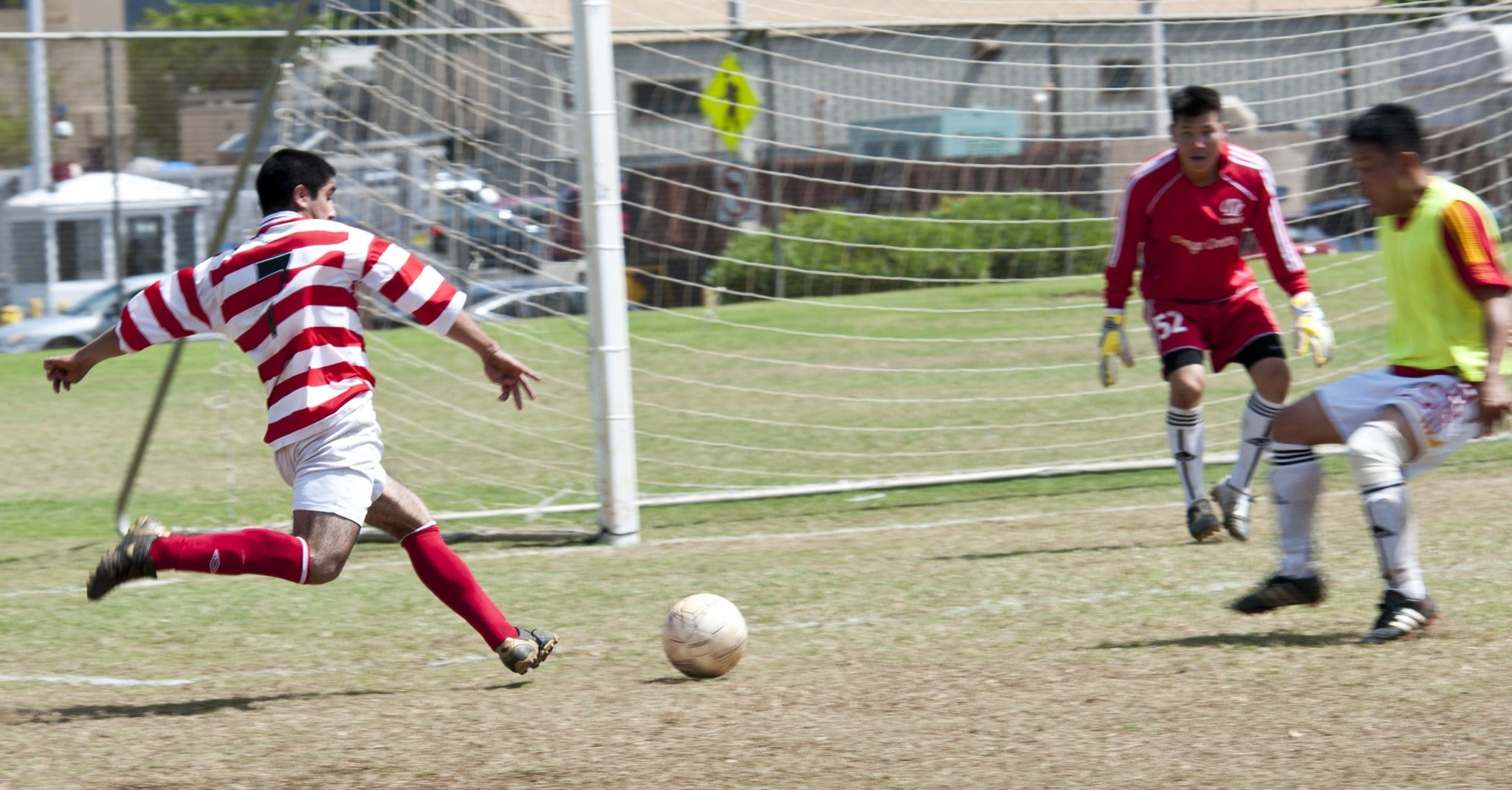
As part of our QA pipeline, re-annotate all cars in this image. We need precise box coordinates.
[1,274,168,353]
[1253,194,1512,257]
[331,163,643,328]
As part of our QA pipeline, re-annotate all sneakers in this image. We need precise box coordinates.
[1362,586,1436,642]
[1236,573,1321,613]
[1186,499,1219,543]
[1212,475,1250,542]
[87,517,172,600]
[497,627,559,674]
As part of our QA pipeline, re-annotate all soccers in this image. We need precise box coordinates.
[663,593,749,680]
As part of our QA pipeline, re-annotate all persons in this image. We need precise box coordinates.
[1228,103,1512,642]
[43,148,559,675]
[1097,85,1335,544]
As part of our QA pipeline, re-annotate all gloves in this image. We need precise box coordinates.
[1097,314,1134,387]
[1290,292,1336,368]
